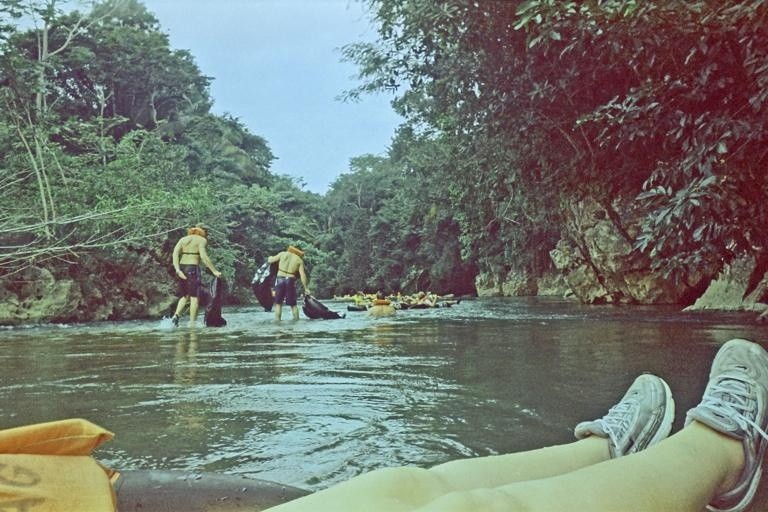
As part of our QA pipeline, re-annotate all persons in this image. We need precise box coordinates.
[329,286,463,315]
[0,336,768,509]
[166,332,213,440]
[266,241,310,320]
[170,221,223,332]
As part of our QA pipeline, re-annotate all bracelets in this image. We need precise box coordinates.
[175,270,181,276]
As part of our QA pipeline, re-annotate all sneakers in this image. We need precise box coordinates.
[172,315,179,326]
[575,373,675,460]
[685,338,768,512]
